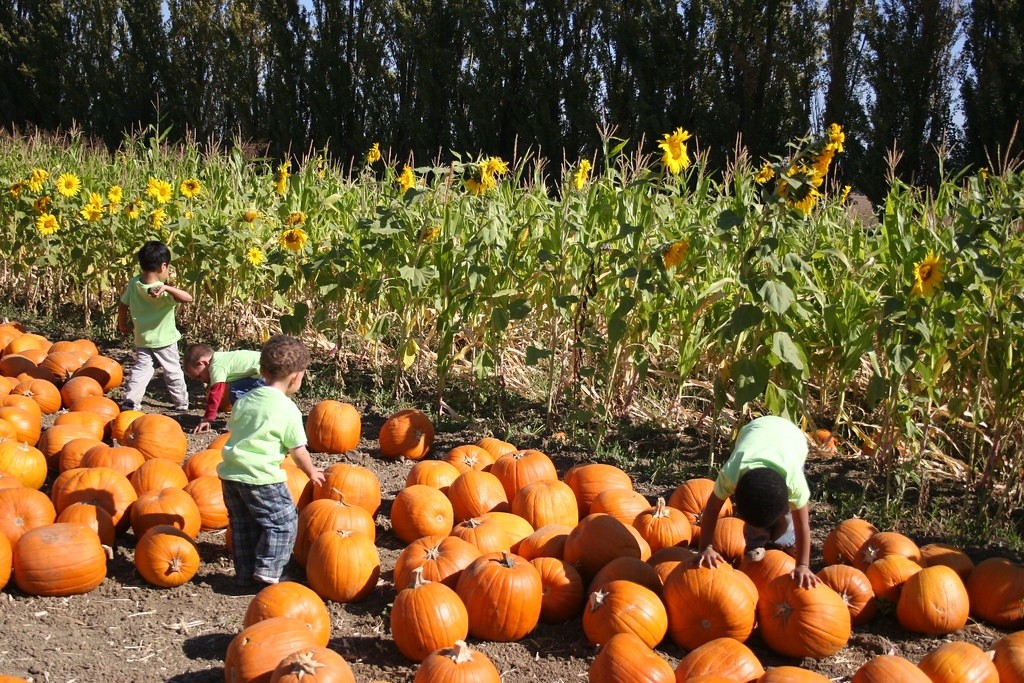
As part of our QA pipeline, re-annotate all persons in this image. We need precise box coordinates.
[183,345,267,433]
[217,335,326,585]
[118,242,192,411]
[695,415,824,591]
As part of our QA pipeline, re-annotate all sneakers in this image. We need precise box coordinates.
[253,571,293,584]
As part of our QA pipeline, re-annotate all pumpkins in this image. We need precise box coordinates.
[0,319,1023,683]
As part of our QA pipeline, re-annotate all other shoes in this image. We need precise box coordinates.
[175,404,189,411]
[744,546,766,562]
[795,542,824,565]
[121,401,142,410]
